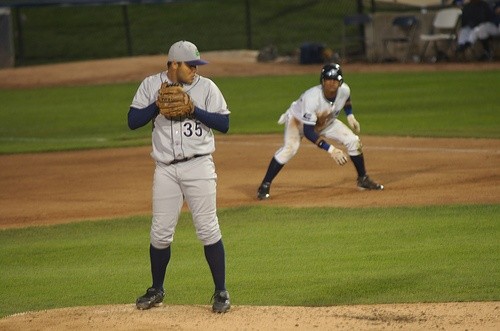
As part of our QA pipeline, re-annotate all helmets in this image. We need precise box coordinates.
[320,63,343,86]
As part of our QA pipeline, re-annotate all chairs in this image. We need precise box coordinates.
[380,15,419,63]
[420,8,463,62]
[342,13,375,64]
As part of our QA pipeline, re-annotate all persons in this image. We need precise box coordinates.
[455,0,500,61]
[256,63,385,199]
[128,41,230,312]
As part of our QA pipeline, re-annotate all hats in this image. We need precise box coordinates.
[167,40,207,65]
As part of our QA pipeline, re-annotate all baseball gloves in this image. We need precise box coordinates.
[158,86,193,120]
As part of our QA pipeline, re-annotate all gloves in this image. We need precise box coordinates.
[327,145,349,166]
[347,113,360,132]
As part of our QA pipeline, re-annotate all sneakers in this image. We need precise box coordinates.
[136,287,164,310]
[357,176,384,189]
[209,290,230,313]
[258,183,270,199]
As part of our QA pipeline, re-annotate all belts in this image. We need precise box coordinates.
[170,153,209,164]
